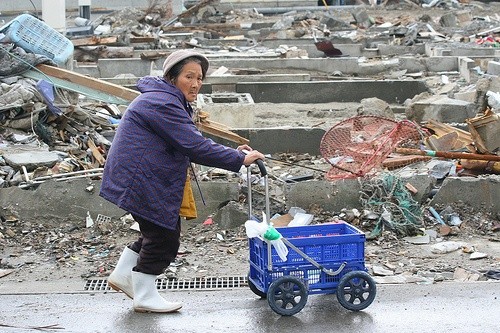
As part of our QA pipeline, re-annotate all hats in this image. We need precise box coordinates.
[163,48,209,77]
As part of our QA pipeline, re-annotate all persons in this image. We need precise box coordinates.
[99,49,265,313]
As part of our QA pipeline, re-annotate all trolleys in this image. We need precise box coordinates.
[241,148,378,318]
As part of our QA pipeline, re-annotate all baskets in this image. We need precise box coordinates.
[0,13,74,68]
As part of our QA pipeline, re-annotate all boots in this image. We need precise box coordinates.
[131,265,182,313]
[107,245,140,301]
